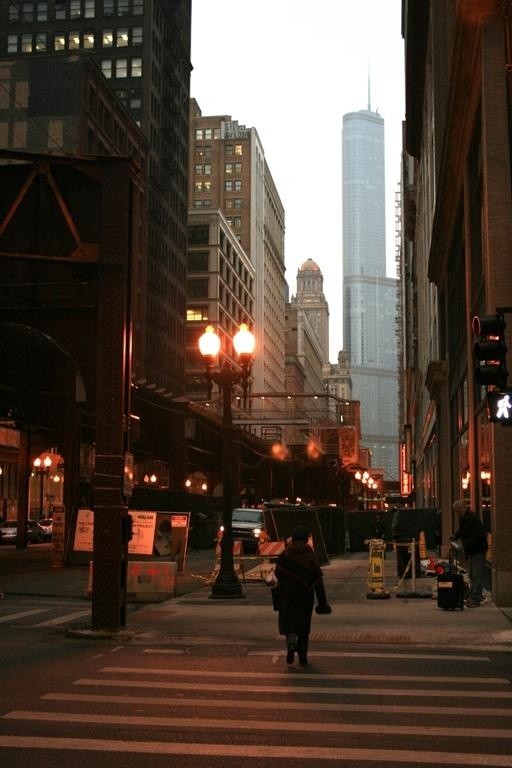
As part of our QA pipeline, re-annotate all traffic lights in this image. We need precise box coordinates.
[472,307,512,427]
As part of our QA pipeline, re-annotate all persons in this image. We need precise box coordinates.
[274,525,328,666]
[369,511,386,540]
[447,500,488,608]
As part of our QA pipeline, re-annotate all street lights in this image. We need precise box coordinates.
[199,322,259,600]
[33,456,51,518]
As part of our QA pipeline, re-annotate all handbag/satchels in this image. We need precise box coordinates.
[270,587,288,611]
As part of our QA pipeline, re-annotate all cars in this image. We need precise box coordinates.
[0,518,54,543]
[231,508,267,549]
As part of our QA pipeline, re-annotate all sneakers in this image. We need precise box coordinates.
[465,601,480,607]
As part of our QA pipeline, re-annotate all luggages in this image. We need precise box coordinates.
[437,548,464,611]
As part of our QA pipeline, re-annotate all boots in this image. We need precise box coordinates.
[287,644,295,663]
[297,650,308,665]
